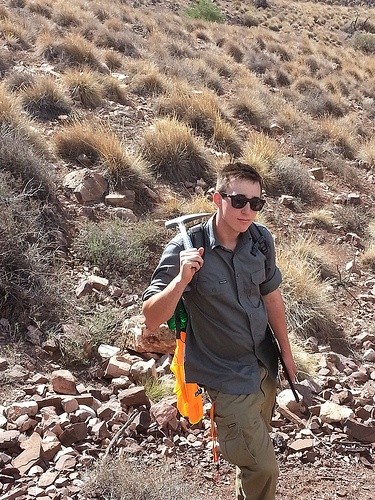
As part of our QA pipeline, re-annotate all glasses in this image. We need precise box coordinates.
[220,192,267,211]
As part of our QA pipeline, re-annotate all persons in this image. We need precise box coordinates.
[142,162,298,500]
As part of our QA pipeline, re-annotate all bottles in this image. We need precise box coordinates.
[167,299,188,331]
